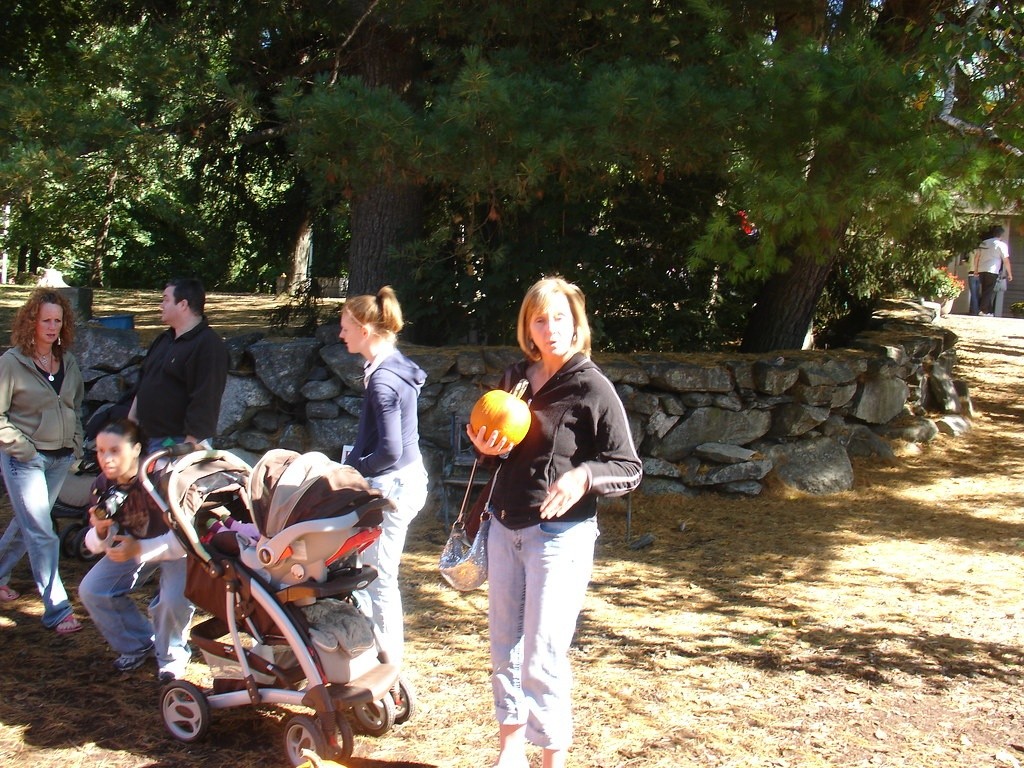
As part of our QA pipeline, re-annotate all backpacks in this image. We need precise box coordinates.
[74,402,130,475]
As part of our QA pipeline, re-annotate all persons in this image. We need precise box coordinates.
[127,276,230,456]
[0,288,85,634]
[465,276,643,768]
[338,286,429,669]
[973,226,1013,317]
[206,515,262,548]
[78,418,196,682]
[967,248,982,316]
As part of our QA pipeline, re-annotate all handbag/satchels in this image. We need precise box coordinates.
[462,361,523,544]
[438,455,502,592]
[992,274,1007,291]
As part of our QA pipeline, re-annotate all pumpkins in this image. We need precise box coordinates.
[470,378,532,448]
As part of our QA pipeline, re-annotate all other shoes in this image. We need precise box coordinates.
[978,311,994,317]
[388,685,403,706]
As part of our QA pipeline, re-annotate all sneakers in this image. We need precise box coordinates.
[114,642,155,671]
[158,652,192,682]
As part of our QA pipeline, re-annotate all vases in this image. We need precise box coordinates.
[928,294,954,314]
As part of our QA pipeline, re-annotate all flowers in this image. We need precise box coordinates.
[926,268,965,301]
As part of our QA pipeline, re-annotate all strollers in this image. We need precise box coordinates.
[138,441,416,768]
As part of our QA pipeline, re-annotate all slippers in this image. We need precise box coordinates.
[0,585,19,600]
[55,613,82,632]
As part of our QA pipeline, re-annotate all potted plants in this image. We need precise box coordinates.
[1010,302,1024,318]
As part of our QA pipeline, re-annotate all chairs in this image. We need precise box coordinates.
[439,412,492,535]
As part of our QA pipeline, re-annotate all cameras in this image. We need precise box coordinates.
[91,483,129,524]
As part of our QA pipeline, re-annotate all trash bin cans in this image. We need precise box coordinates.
[992,276,1007,317]
[97,313,137,329]
